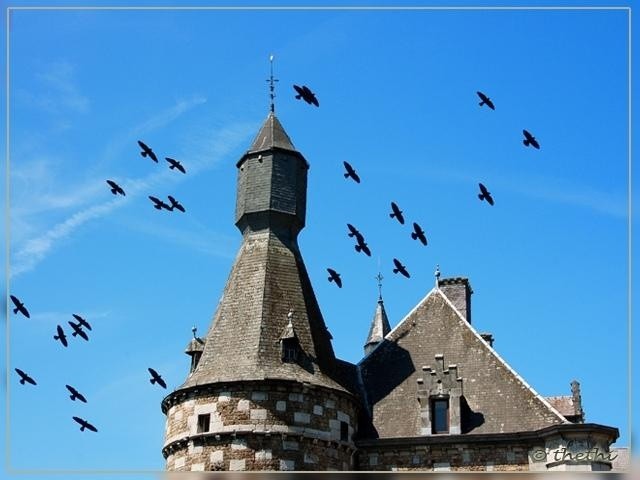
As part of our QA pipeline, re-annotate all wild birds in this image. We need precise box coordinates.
[393,258,411,279]
[478,183,494,206]
[72,416,98,432]
[389,202,427,247]
[105,179,126,197]
[343,160,360,183]
[148,368,167,388]
[346,223,371,257]
[522,129,540,149]
[477,91,495,110]
[14,368,37,385]
[137,140,186,174]
[65,384,87,403]
[147,193,186,212]
[53,314,92,348]
[9,294,30,318]
[293,84,320,107]
[327,268,343,289]
